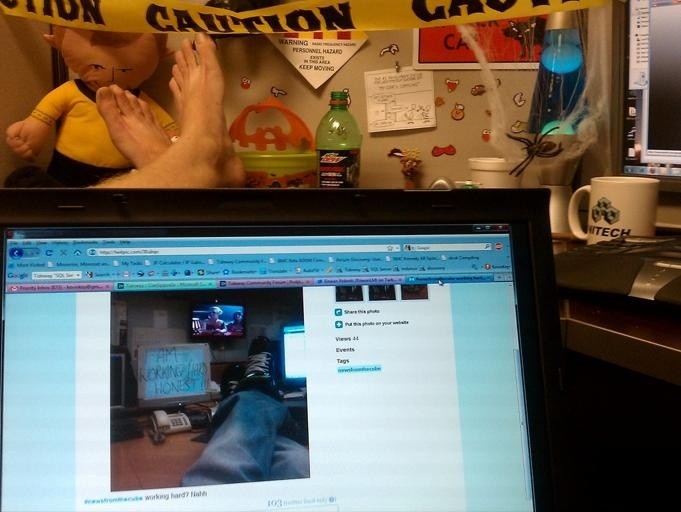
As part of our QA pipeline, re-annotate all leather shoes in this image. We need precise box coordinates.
[220,362,245,399]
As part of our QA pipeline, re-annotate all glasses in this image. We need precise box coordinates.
[210,312,215,313]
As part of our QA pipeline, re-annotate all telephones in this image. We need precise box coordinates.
[148,409,192,444]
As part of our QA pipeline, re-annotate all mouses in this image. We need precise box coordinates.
[190,415,207,429]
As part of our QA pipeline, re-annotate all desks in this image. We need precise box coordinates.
[559,293,681,387]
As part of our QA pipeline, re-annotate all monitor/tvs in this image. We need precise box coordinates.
[138,343,211,412]
[0,186,558,512]
[279,323,307,398]
[111,345,127,410]
[189,303,247,340]
[620,0,680,180]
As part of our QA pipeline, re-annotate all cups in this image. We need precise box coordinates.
[567,176,660,246]
[237,151,318,189]
[466,159,525,189]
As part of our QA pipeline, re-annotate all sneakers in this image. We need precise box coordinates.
[244,335,273,387]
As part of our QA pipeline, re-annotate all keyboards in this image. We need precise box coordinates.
[111,417,145,442]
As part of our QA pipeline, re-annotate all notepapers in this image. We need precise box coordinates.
[361,66,438,133]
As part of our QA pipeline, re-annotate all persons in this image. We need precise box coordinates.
[227,311,243,332]
[201,306,226,335]
[84,32,246,189]
[181,335,310,486]
[6,23,179,189]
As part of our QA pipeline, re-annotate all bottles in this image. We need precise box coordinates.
[313,87,363,189]
